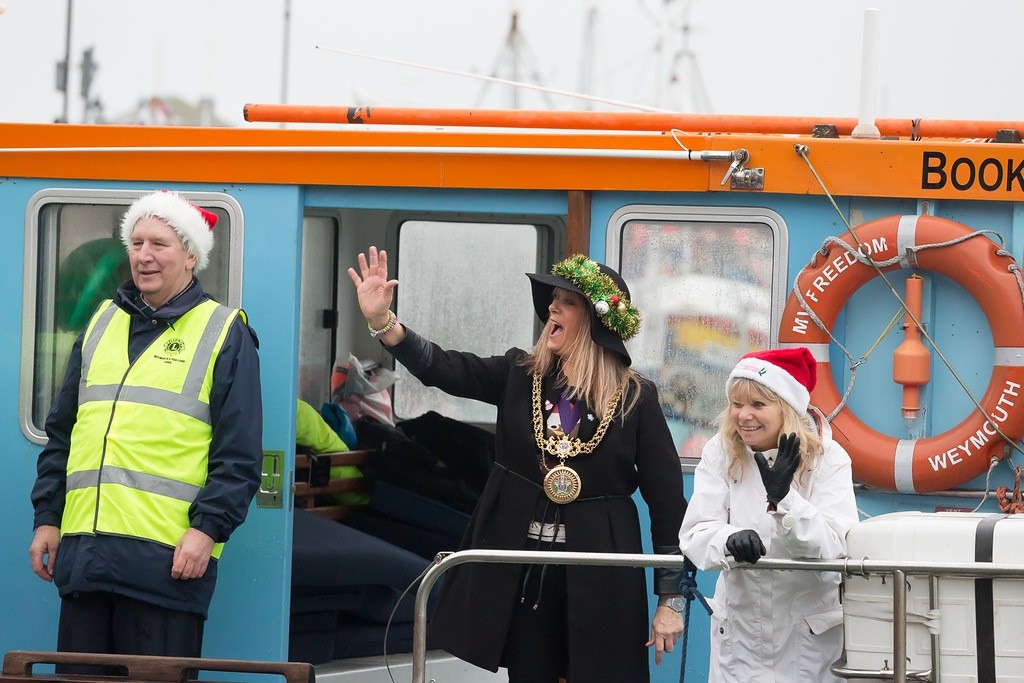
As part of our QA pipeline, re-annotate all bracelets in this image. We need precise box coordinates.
[368,310,397,339]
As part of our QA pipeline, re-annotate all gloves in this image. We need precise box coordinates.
[726,530,767,566]
[753,432,802,505]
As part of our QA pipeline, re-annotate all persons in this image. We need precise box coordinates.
[296,359,397,508]
[349,243,691,683]
[28,189,264,679]
[678,349,857,683]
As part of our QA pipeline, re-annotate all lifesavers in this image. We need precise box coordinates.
[782,213,1023,494]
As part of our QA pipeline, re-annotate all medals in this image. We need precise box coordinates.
[542,465,582,504]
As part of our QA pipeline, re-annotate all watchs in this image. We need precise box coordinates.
[658,595,687,612]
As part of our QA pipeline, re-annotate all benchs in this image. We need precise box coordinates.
[284,451,448,664]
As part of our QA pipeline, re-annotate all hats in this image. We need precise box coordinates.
[524,254,632,368]
[120,189,218,272]
[725,347,817,418]
